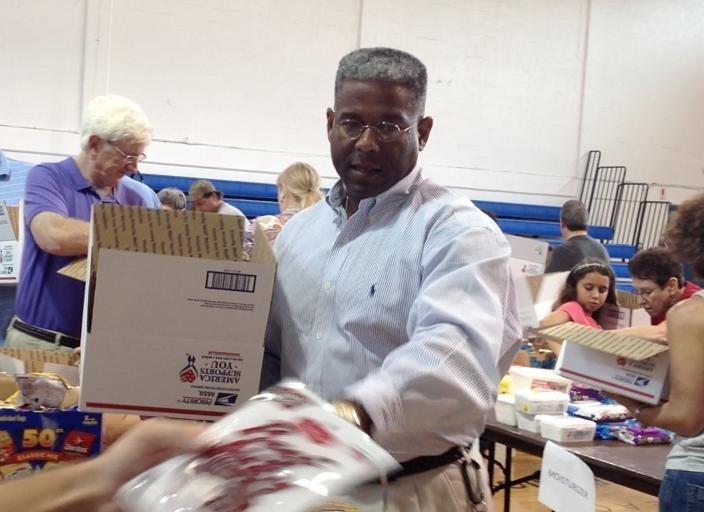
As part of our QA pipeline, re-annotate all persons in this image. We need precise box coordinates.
[2,417,215,512]
[627,246,702,325]
[243,162,326,262]
[3,95,161,367]
[540,256,620,360]
[157,187,187,212]
[264,48,522,512]
[543,200,610,274]
[599,195,703,512]
[187,180,250,248]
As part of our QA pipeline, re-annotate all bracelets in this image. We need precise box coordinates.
[633,404,647,421]
[331,399,364,429]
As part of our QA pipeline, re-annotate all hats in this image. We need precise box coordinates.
[186,178,225,203]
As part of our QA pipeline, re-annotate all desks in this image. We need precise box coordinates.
[477,407,675,511]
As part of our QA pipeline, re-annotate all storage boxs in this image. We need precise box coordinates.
[76,198,279,423]
[505,234,674,410]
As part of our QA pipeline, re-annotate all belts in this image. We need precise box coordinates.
[359,443,465,487]
[12,316,79,348]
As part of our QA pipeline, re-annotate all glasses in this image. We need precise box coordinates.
[113,143,146,166]
[332,114,416,143]
